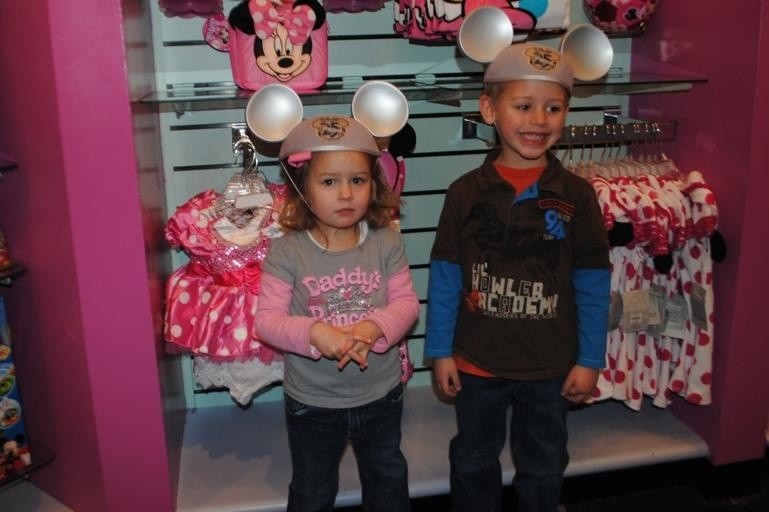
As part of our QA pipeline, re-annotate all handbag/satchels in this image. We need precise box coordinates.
[228,1,329,92]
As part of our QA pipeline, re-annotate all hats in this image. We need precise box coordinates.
[456,6,614,95]
[245,80,409,161]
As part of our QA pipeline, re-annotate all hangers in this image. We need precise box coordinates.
[212,134,281,221]
[557,120,680,185]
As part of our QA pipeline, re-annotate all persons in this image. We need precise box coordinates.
[253,113,424,512]
[424,43,611,511]
